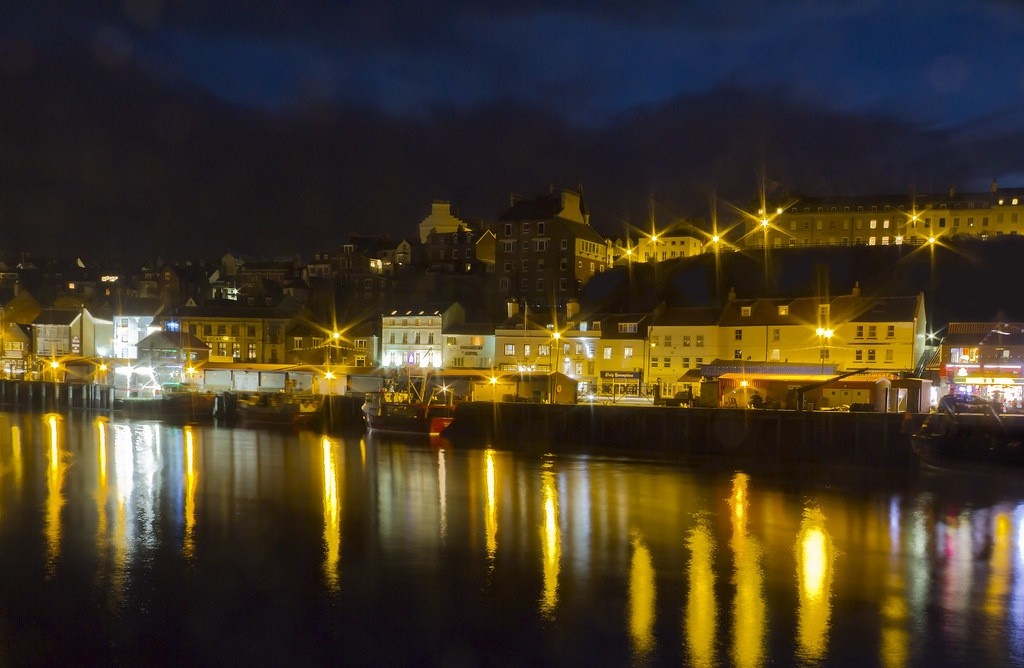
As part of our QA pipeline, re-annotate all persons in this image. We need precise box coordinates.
[948,402,960,420]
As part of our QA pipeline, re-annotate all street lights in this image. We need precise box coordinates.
[550,333,561,404]
[816,327,835,373]
[643,337,656,382]
